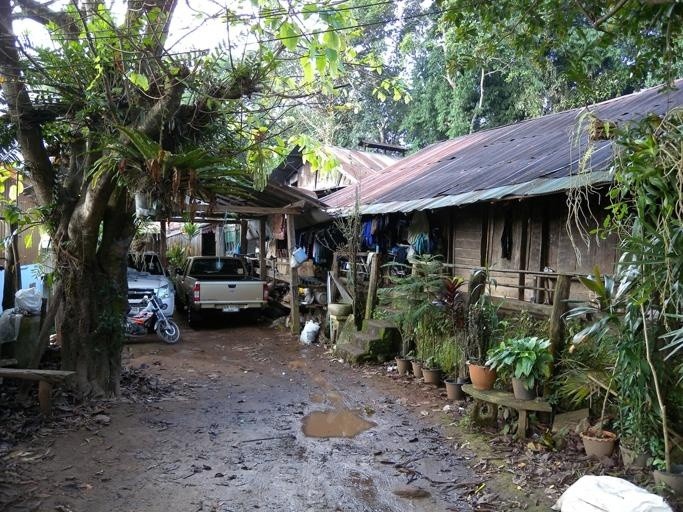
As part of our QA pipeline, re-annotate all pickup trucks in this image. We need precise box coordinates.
[175,256,270,329]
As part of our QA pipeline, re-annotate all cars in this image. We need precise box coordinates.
[127,251,176,318]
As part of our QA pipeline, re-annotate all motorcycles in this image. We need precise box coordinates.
[123,290,181,344]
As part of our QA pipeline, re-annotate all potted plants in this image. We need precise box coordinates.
[378,252,503,401]
[560,252,661,471]
[485,337,555,399]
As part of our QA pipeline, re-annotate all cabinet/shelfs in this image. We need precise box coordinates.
[255,267,326,310]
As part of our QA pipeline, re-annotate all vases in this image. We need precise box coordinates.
[328,303,352,316]
[654,462,683,494]
[579,428,616,459]
